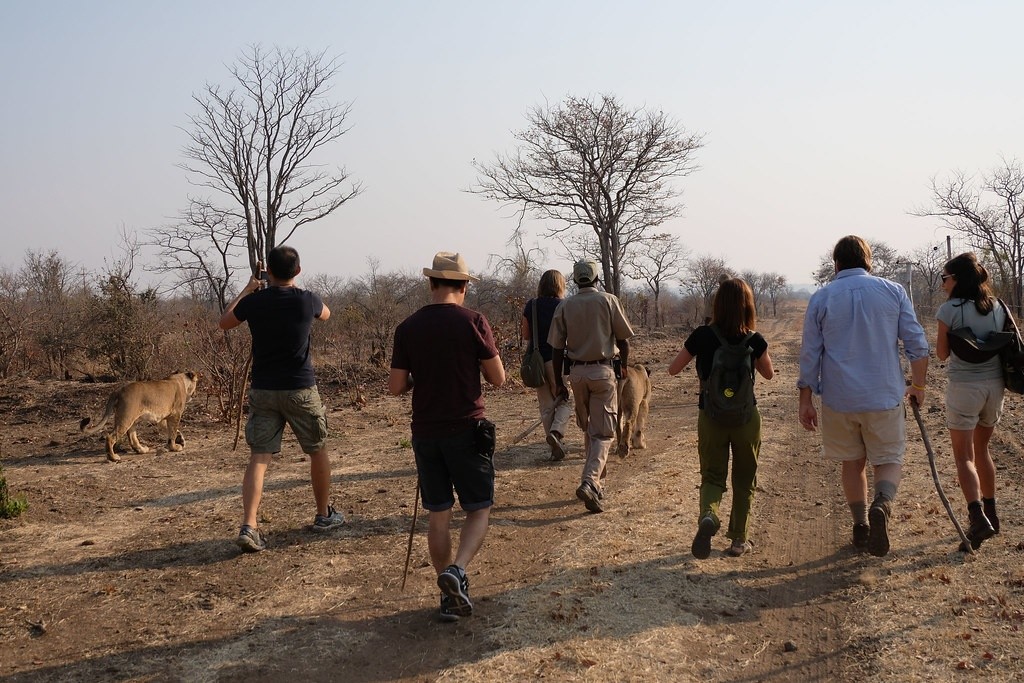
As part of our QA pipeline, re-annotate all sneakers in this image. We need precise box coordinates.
[986,512,1000,536]
[852,523,869,550]
[546,433,566,462]
[868,498,890,557]
[438,591,462,622]
[436,565,473,615]
[575,481,605,513]
[730,539,755,556]
[313,504,344,532]
[691,517,717,559]
[236,524,267,550]
[959,511,994,552]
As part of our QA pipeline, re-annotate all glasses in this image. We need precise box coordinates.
[941,272,956,284]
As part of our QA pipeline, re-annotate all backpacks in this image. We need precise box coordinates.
[700,318,758,427]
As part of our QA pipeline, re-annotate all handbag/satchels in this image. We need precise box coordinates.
[997,296,1024,395]
[521,349,544,387]
[470,418,497,461]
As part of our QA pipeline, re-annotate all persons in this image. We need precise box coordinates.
[936,253,1006,551]
[667,280,773,560]
[220,247,345,548]
[706,275,729,326]
[546,259,634,515]
[519,270,573,460]
[796,235,928,556]
[390,252,505,624]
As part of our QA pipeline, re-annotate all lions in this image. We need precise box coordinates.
[80,371,198,462]
[615,364,651,459]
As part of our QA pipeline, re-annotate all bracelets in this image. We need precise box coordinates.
[911,383,925,391]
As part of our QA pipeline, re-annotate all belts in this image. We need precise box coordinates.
[570,360,609,365]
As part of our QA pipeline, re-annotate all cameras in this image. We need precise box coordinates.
[261,271,270,281]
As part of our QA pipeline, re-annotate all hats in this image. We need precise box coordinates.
[574,259,599,284]
[424,253,481,281]
